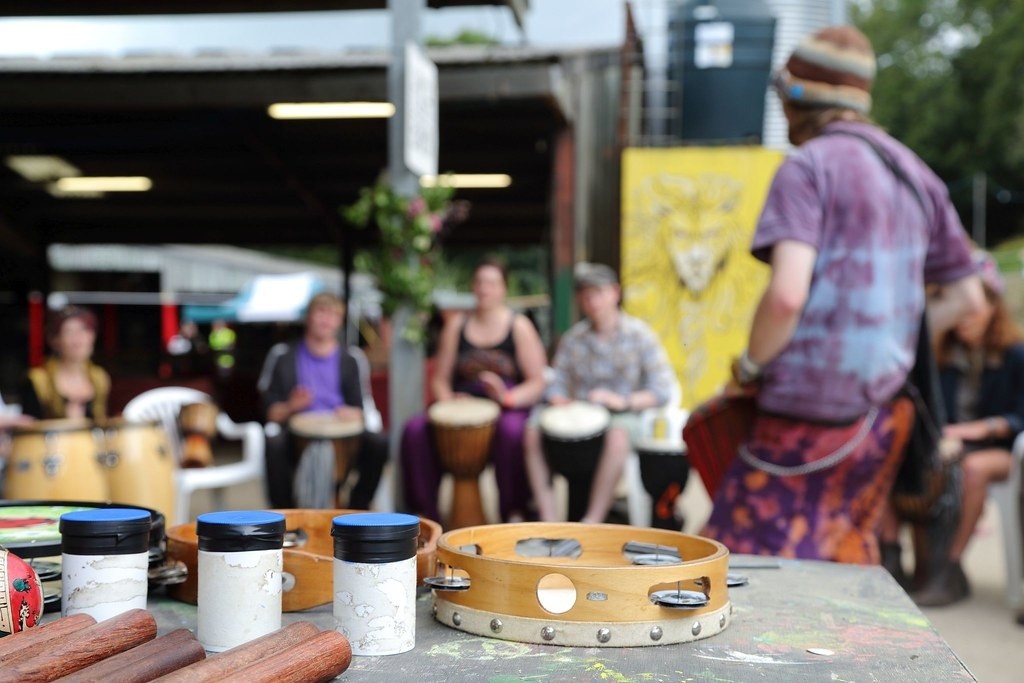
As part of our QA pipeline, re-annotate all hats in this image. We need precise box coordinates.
[774,26,877,112]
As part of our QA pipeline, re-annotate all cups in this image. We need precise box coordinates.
[195,510,286,654]
[59,508,152,624]
[334,513,420,655]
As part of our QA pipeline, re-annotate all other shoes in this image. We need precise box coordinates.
[911,565,971,606]
[876,536,909,591]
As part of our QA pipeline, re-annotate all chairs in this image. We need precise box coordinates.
[615,378,688,535]
[124,388,262,518]
[989,428,1024,621]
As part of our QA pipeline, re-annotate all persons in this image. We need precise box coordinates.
[171,318,237,418]
[523,263,674,525]
[23,306,112,418]
[248,293,388,509]
[401,259,548,524]
[693,24,1024,610]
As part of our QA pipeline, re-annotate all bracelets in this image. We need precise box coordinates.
[730,355,763,386]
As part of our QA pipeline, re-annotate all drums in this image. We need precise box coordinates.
[423,519,744,647]
[632,446,691,531]
[0,419,106,502]
[95,416,172,531]
[1,498,186,633]
[149,509,444,611]
[426,396,502,527]
[287,408,364,509]
[538,399,610,522]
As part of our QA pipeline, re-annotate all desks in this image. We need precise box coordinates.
[32,547,980,683]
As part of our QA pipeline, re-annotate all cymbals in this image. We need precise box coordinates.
[649,587,710,612]
[631,548,681,569]
[726,573,747,591]
[147,553,187,589]
[26,560,63,605]
[426,571,473,594]
[280,525,308,548]
